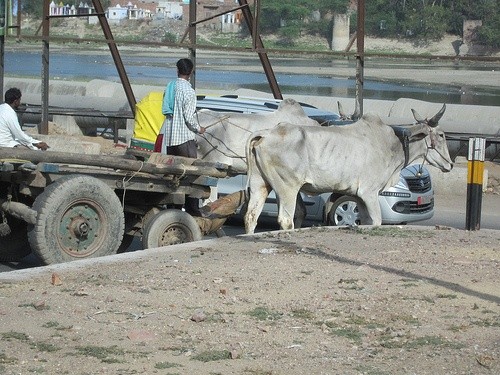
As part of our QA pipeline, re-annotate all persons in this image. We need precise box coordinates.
[0,87,50,151]
[162,56,206,217]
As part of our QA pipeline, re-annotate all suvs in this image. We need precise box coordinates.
[155,94,436,227]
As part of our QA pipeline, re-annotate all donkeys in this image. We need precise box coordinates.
[244,105,453,233]
[193,98,360,229]
